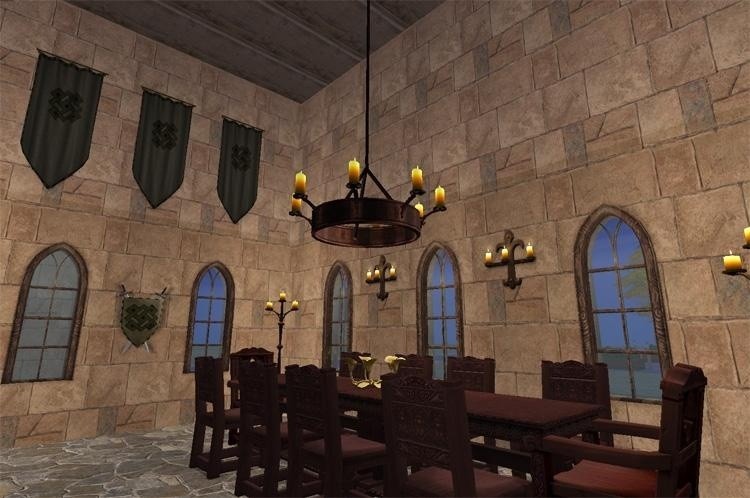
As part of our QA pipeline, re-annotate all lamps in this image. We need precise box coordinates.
[264,291,300,372]
[721,227,750,283]
[484,231,536,288]
[365,255,397,301]
[289,24,446,248]
